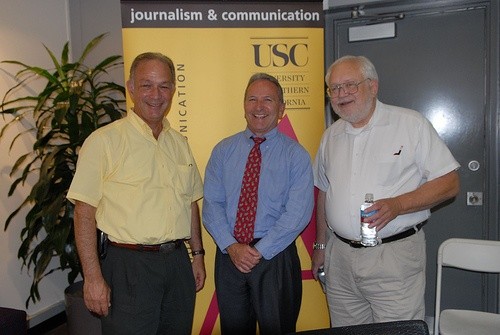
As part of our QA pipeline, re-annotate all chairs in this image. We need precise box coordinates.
[434,238,500,335]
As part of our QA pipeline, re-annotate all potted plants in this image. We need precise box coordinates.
[0,30,125,335]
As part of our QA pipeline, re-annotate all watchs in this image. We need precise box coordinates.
[192,248,205,255]
[313,242,326,250]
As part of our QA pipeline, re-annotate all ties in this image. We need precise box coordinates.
[233,136,264,244]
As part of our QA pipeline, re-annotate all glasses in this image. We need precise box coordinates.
[326,80,366,98]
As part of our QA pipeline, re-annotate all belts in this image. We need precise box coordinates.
[334,219,429,248]
[112,241,182,253]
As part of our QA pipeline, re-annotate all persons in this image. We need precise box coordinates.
[311,55,461,328]
[66,52,207,335]
[202,73,315,335]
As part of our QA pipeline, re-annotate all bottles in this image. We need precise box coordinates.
[360,193,378,247]
[319,265,326,293]
